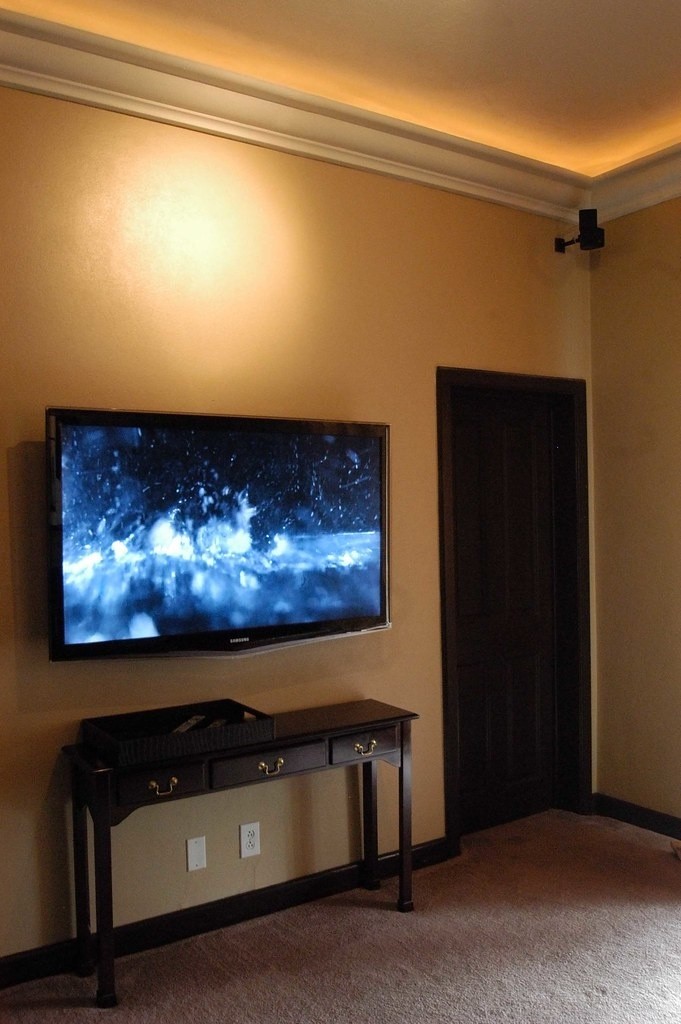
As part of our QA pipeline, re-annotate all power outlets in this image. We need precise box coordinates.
[238,821,261,859]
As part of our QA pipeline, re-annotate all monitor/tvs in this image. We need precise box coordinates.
[45,403,393,663]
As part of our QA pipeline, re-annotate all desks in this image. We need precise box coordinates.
[72,692,423,1007]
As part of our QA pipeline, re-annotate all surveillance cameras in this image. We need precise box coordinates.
[579,208,604,251]
